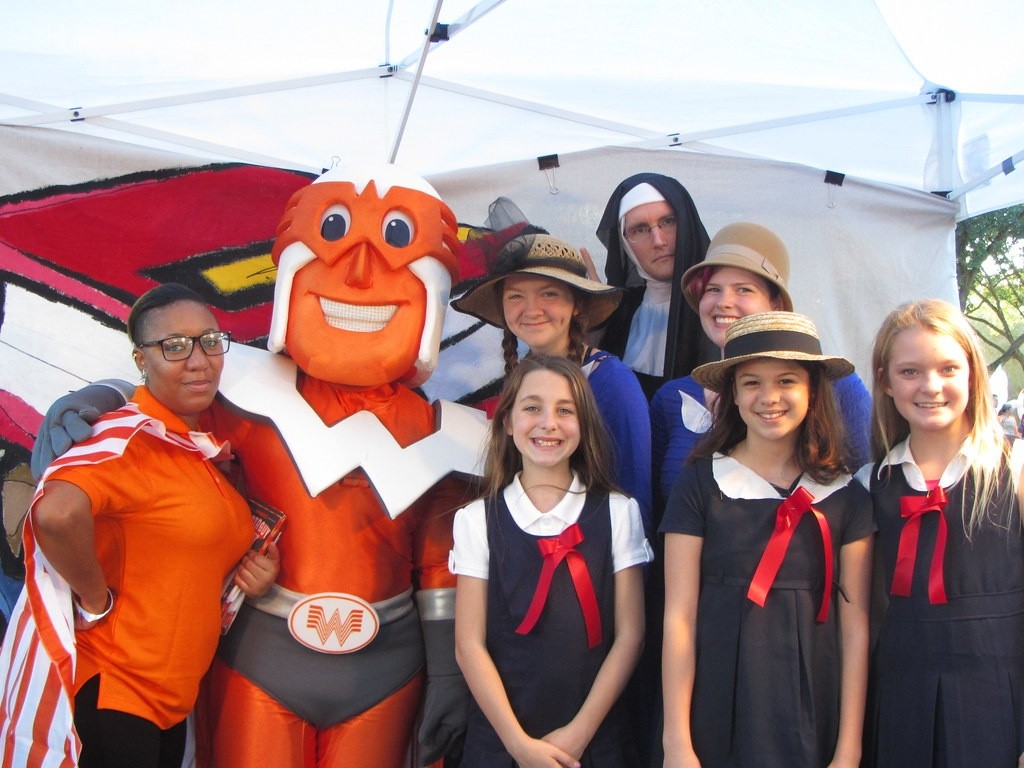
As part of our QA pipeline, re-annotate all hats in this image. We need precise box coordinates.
[691,310,854,394]
[449,224,627,334]
[679,222,793,316]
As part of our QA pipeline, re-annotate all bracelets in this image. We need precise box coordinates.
[73,587,113,622]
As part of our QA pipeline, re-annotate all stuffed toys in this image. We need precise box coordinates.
[32,164,494,767]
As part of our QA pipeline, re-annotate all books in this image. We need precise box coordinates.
[220,499,286,637]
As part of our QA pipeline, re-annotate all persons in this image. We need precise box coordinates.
[450,173,1024,768]
[0,283,281,768]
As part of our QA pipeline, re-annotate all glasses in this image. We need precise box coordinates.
[626,214,679,244]
[139,331,233,362]
